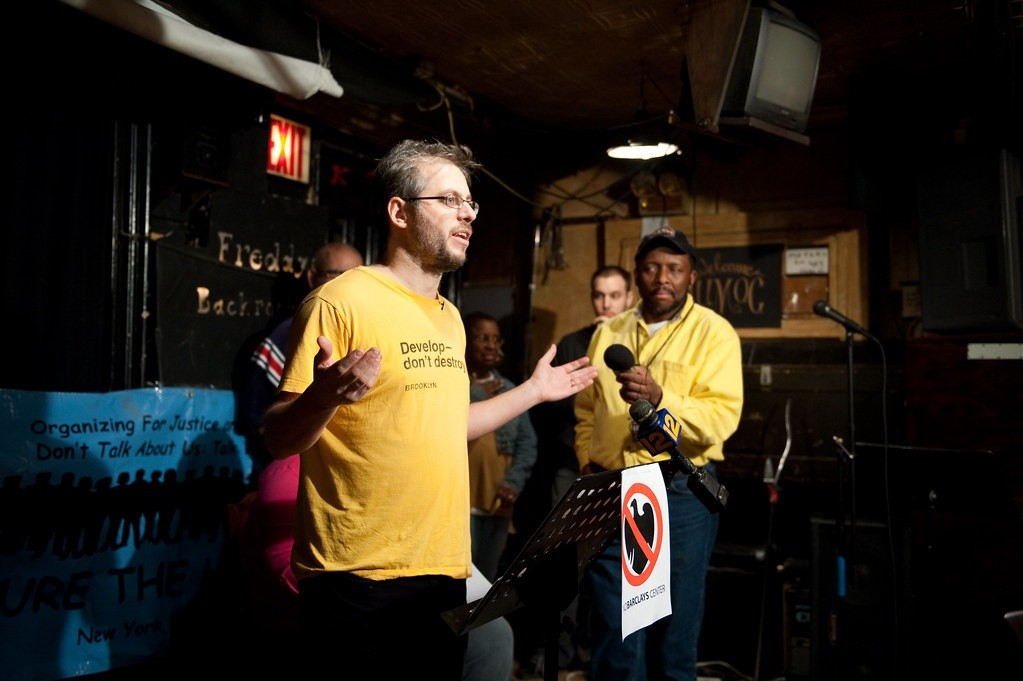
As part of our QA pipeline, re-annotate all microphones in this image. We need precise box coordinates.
[629,397,697,475]
[813,300,879,343]
[603,344,637,372]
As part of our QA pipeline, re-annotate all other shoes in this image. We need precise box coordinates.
[517,663,542,679]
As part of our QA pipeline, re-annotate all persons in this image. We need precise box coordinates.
[261,141,599,681]
[235,231,745,680]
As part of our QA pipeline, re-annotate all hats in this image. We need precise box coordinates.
[634,227,689,261]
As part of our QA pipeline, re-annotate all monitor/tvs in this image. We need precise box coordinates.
[723,9,822,134]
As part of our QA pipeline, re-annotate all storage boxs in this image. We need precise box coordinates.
[711,363,888,559]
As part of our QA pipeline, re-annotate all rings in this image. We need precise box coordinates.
[571,379,574,386]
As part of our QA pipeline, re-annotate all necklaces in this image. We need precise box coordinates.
[635,304,696,368]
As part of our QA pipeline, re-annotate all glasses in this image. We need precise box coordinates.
[469,334,503,348]
[315,269,344,280]
[402,197,479,215]
